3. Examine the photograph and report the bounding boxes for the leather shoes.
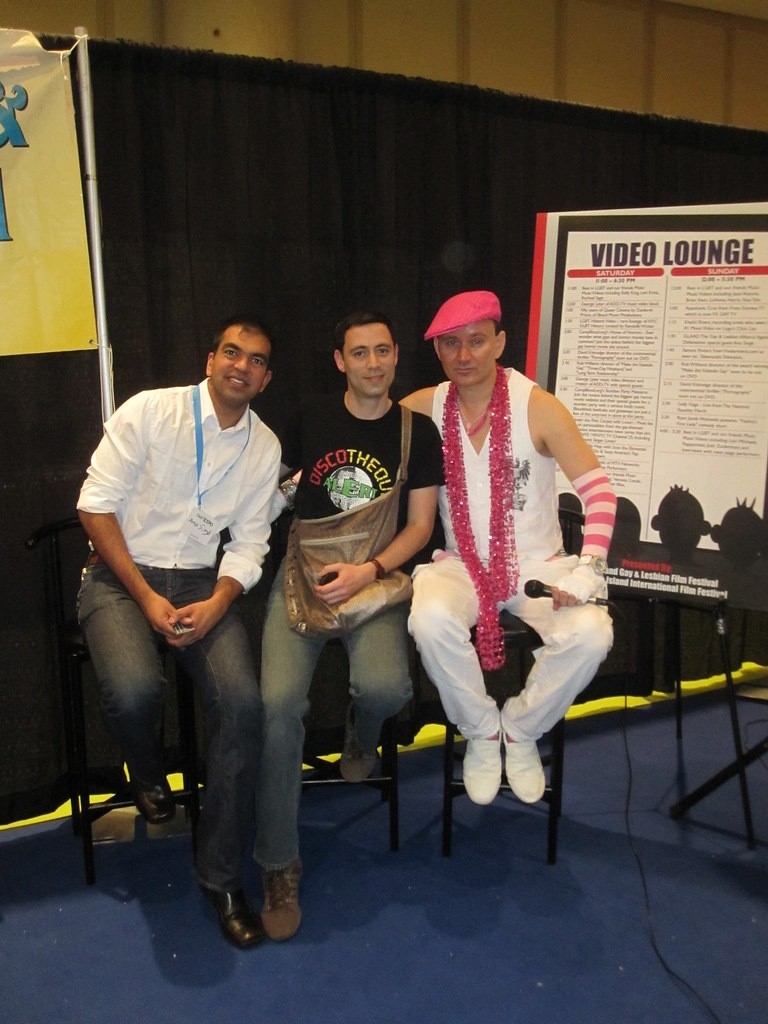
[133,780,175,824]
[462,712,546,805]
[203,888,265,950]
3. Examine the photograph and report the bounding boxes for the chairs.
[29,518,586,887]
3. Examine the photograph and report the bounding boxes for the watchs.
[579,555,607,574]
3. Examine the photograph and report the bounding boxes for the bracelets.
[371,559,384,578]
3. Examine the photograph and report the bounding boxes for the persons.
[254,311,442,941]
[268,291,618,805]
[77,312,283,948]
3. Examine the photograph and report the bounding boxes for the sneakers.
[340,701,377,783]
[260,857,302,941]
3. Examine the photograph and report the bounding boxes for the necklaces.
[443,363,520,671]
[456,397,490,436]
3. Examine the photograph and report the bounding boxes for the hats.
[424,291,501,340]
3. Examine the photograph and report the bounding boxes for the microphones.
[524,580,615,607]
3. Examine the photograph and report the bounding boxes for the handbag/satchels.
[283,404,416,638]
